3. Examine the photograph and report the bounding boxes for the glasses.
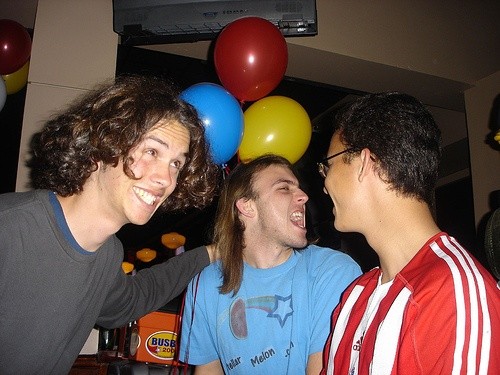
[317,148,376,178]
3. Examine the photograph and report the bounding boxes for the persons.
[178,153,363,375]
[0,73,221,375]
[316,90,500,375]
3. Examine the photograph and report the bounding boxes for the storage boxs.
[129,311,187,367]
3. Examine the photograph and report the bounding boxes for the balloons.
[238,94,313,166]
[177,81,245,170]
[0,18,32,112]
[213,16,290,103]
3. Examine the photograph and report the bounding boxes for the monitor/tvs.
[112,0,318,45]
